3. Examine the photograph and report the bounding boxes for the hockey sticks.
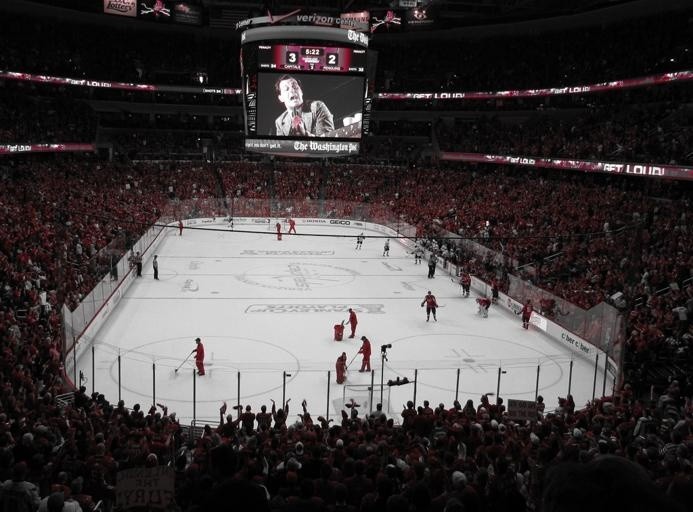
[175,350,195,373]
[345,349,361,370]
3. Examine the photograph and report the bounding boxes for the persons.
[1,81,689,512]
[270,74,338,142]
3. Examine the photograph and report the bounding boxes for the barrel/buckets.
[334,323,344,342]
[278,232,282,241]
